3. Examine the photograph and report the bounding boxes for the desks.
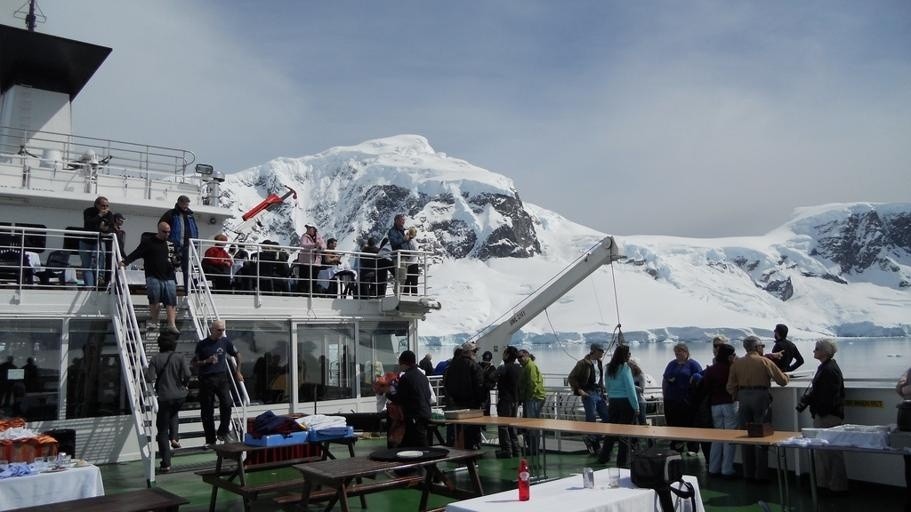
[0,403,910,512]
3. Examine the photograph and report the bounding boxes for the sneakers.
[167,326,180,335]
[513,450,521,458]
[495,450,512,458]
[218,433,236,443]
[202,444,217,451]
[159,466,171,471]
[149,323,161,329]
[526,449,538,455]
[171,440,181,449]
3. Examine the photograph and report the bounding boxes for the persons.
[803,338,848,496]
[599,345,640,468]
[250,340,385,405]
[0,345,132,422]
[117,221,180,333]
[625,353,653,448]
[897,368,910,504]
[193,319,244,450]
[664,344,703,456]
[375,338,543,474]
[727,336,789,489]
[143,334,191,473]
[0,196,421,298]
[713,338,724,365]
[773,324,804,372]
[704,344,744,481]
[568,343,609,454]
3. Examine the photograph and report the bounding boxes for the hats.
[590,342,604,350]
[399,351,415,367]
[305,223,317,228]
[464,343,479,350]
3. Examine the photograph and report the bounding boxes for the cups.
[608,464,621,487]
[58,452,66,465]
[308,425,317,440]
[34,455,57,467]
[347,425,355,435]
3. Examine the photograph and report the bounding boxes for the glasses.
[162,231,170,233]
[755,344,766,349]
[96,203,108,207]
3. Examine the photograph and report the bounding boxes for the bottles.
[519,459,530,502]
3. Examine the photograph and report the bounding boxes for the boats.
[488,369,666,419]
[1,0,910,511]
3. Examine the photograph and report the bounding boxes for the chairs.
[0,225,361,298]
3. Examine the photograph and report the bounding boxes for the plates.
[73,463,92,467]
[396,450,423,458]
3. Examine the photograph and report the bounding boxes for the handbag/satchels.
[631,448,683,489]
